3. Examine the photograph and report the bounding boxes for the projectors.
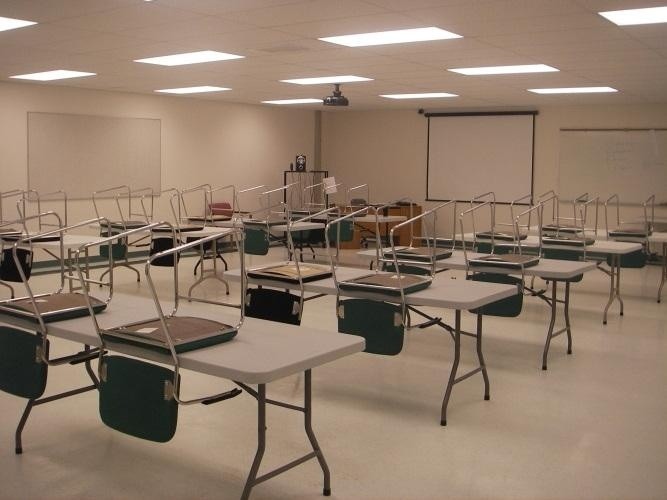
[323,91,349,107]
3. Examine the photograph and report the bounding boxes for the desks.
[183,213,325,275]
[277,210,409,271]
[0,286,366,499]
[6,231,116,301]
[570,225,663,304]
[222,260,519,426]
[357,247,596,371]
[420,232,641,324]
[100,215,234,302]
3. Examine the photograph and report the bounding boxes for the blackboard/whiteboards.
[555,128,667,208]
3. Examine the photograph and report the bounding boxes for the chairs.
[539,191,588,237]
[458,201,543,317]
[1,179,369,261]
[538,197,600,280]
[375,195,457,278]
[468,193,532,254]
[602,194,658,269]
[0,213,111,456]
[326,199,432,355]
[74,222,244,443]
[239,204,340,325]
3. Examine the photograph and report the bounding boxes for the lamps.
[322,83,349,107]
